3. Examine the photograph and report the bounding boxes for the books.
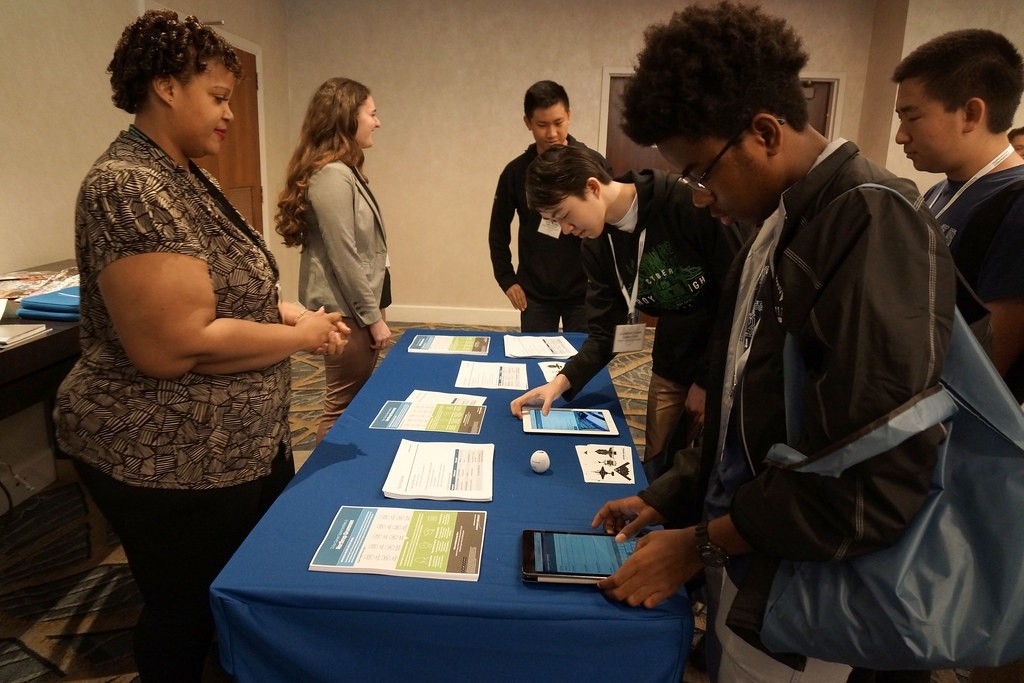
[0,324,54,350]
[17,285,81,321]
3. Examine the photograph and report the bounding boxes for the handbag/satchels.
[725,188,1023,675]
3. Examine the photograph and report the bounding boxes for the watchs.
[694,517,731,571]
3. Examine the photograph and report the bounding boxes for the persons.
[592,1,958,683]
[508,143,747,484]
[892,27,1024,682]
[48,11,355,683]
[1005,125,1023,159]
[274,73,393,447]
[487,79,611,336]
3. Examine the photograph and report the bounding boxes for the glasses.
[677,117,785,197]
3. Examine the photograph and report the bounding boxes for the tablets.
[521,529,645,579]
[521,407,619,436]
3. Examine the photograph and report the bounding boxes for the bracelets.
[293,307,309,323]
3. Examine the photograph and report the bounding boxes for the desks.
[209,329,694,683]
[0,258,110,516]
